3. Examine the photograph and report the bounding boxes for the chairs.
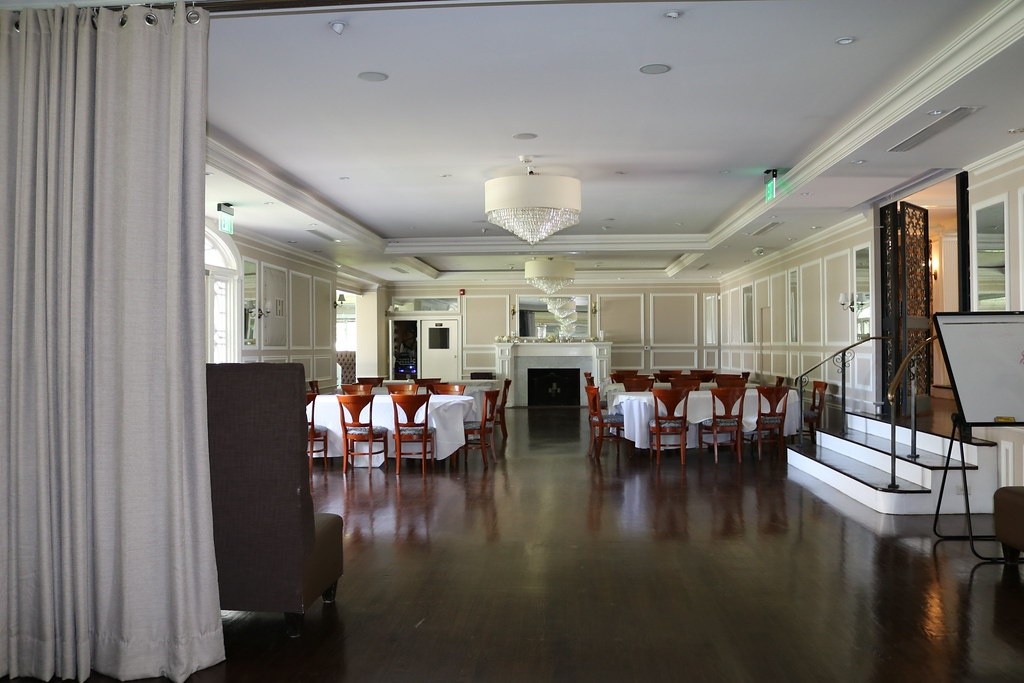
[754,478,789,539]
[708,475,746,540]
[305,368,828,475]
[390,475,438,554]
[587,456,628,534]
[206,364,342,640]
[343,471,389,552]
[653,479,691,543]
[451,463,501,545]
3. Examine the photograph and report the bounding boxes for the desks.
[612,388,805,465]
[603,382,759,436]
[600,377,676,411]
[334,387,491,439]
[381,380,502,411]
[305,394,476,467]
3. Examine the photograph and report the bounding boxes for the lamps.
[538,296,578,338]
[334,294,346,308]
[510,305,516,320]
[484,166,582,245]
[524,256,575,295]
[857,293,866,310]
[591,302,597,316]
[245,301,255,317]
[839,292,855,312]
[258,300,272,319]
[385,305,394,317]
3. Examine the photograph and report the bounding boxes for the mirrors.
[855,241,871,344]
[242,260,256,345]
[516,294,592,340]
[970,192,1010,311]
